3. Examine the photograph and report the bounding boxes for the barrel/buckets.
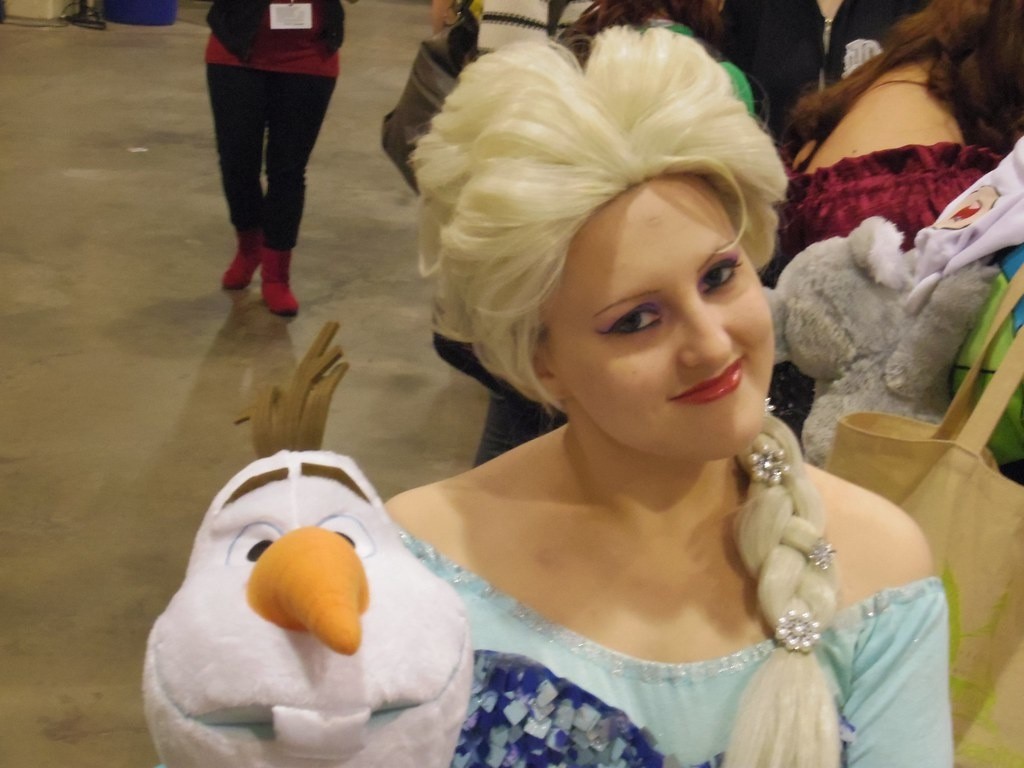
[102,0,178,26]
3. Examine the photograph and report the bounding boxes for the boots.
[222,229,265,289]
[259,244,298,316]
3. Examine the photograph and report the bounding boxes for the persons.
[203,0,351,316]
[382,24,957,768]
[375,0,1024,478]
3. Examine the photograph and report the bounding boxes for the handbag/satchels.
[828,265,1024,768]
[381,0,484,197]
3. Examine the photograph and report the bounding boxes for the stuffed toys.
[762,216,1001,472]
[139,319,471,768]
